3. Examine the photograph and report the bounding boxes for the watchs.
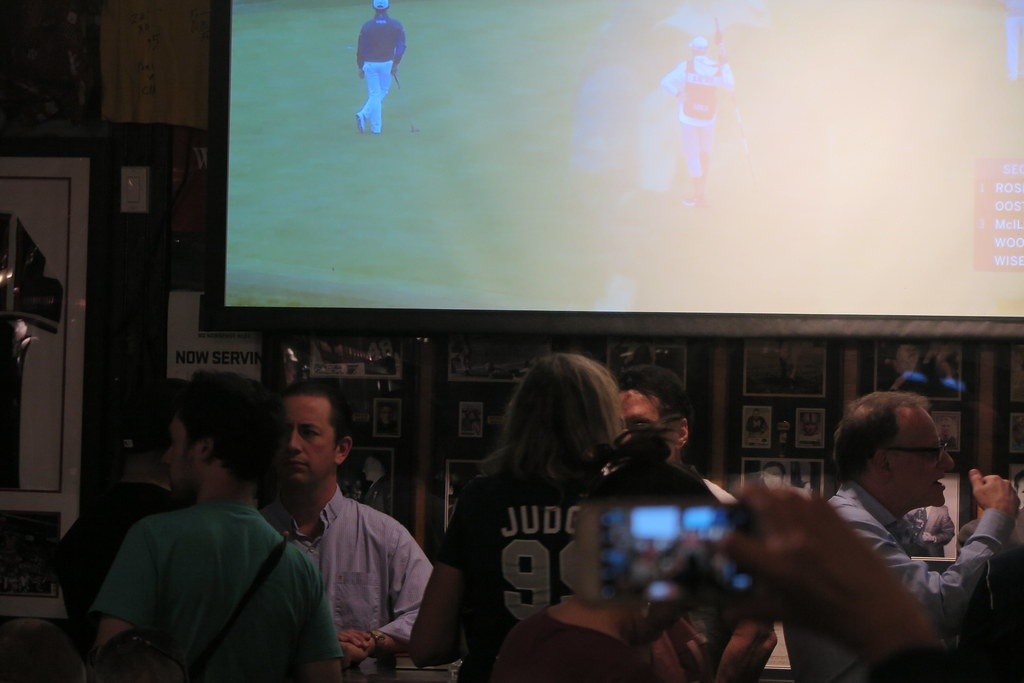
[367,628,386,658]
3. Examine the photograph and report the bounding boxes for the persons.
[461,407,481,435]
[800,411,822,445]
[355,0,405,134]
[747,338,823,393]
[407,352,739,683]
[721,391,1024,683]
[1011,469,1024,546]
[57,369,344,683]
[746,461,803,515]
[889,342,959,399]
[490,423,776,683]
[660,35,735,205]
[378,405,398,434]
[745,408,768,447]
[0,619,190,683]
[258,378,434,666]
[360,454,390,513]
[904,506,954,557]
[939,417,957,447]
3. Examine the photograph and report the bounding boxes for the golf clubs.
[395,73,420,133]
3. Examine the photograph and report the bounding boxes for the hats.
[372,0,389,10]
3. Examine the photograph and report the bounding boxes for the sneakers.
[370,125,380,133]
[356,112,366,133]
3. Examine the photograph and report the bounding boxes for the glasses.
[884,442,948,461]
[88,630,188,683]
[621,415,684,432]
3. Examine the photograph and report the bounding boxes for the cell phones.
[576,502,761,606]
